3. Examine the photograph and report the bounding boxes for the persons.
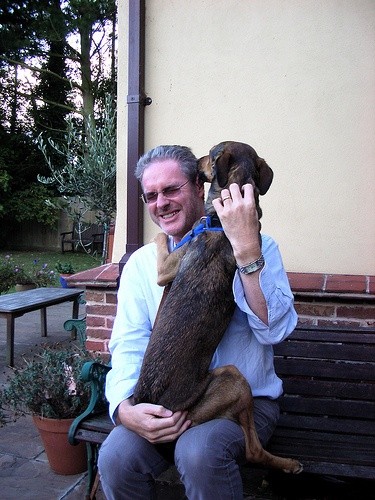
[98,145,298,499]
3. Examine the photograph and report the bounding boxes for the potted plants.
[56,261,78,289]
[0,342,112,477]
[0,255,37,294]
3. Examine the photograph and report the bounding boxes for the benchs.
[63,294,87,354]
[68,328,375,500]
[60,222,104,254]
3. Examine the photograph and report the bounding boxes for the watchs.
[237,254,266,274]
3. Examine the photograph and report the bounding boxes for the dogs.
[131,138,305,479]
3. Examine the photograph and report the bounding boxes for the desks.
[0,287,84,367]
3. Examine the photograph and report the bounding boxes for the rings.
[220,196,232,203]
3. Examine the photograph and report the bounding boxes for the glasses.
[141,179,191,204]
